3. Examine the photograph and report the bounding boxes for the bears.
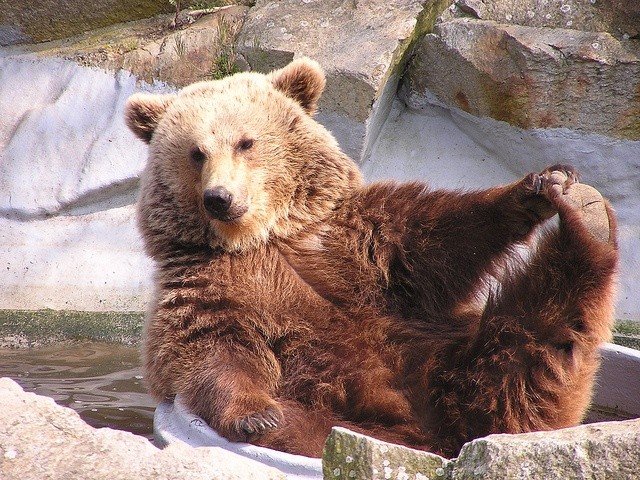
[122,56,620,462]
